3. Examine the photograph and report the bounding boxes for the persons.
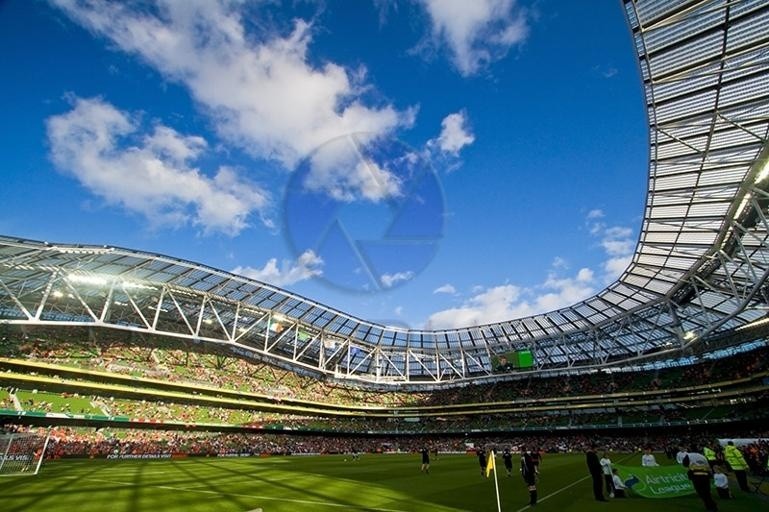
[519,445,541,509]
[712,464,736,500]
[586,443,610,503]
[239,355,442,457]
[476,446,487,478]
[600,452,617,495]
[439,346,768,453]
[351,446,362,461]
[433,445,439,460]
[1,321,252,472]
[641,449,661,467]
[503,448,513,475]
[725,440,753,493]
[422,443,431,473]
[682,443,718,512]
[608,469,631,499]
